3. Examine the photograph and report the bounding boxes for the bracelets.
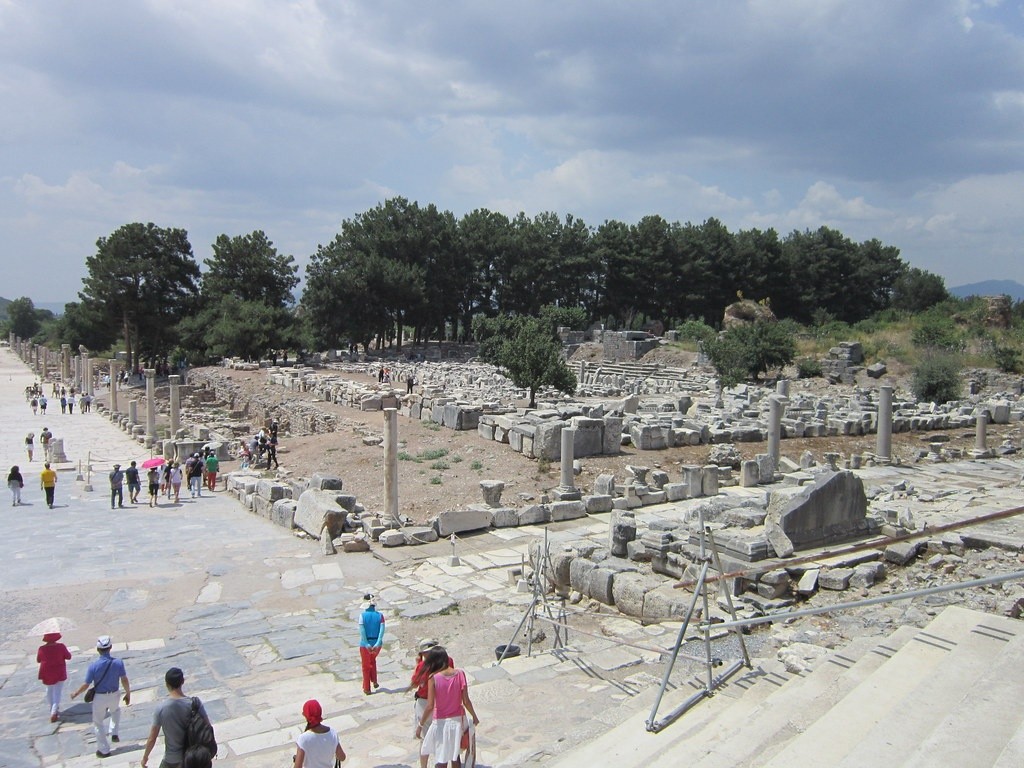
[418,722,423,728]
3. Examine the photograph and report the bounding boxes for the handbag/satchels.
[84,687,95,702]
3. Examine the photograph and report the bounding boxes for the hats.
[360,594,377,612]
[43,633,61,642]
[194,453,200,457]
[96,634,111,649]
[302,700,322,726]
[418,638,435,654]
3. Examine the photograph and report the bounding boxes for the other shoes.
[373,683,379,688]
[111,735,119,742]
[364,690,371,695]
[133,497,138,502]
[95,749,110,757]
[50,715,60,722]
[130,499,134,504]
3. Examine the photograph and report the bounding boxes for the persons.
[350,343,358,356]
[404,637,480,768]
[40,427,53,461]
[70,635,130,759]
[141,667,217,768]
[379,366,391,384]
[109,464,124,510]
[407,369,415,394]
[358,593,385,695]
[51,383,91,415]
[126,461,142,504]
[272,349,288,366]
[7,465,24,506]
[102,361,177,388]
[23,382,48,416]
[24,432,35,463]
[239,419,280,471]
[292,699,346,768]
[146,459,183,508]
[36,633,72,723]
[39,462,58,509]
[186,446,220,499]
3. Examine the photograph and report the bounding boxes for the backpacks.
[179,696,218,768]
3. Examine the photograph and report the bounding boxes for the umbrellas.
[141,458,165,470]
[26,616,79,638]
